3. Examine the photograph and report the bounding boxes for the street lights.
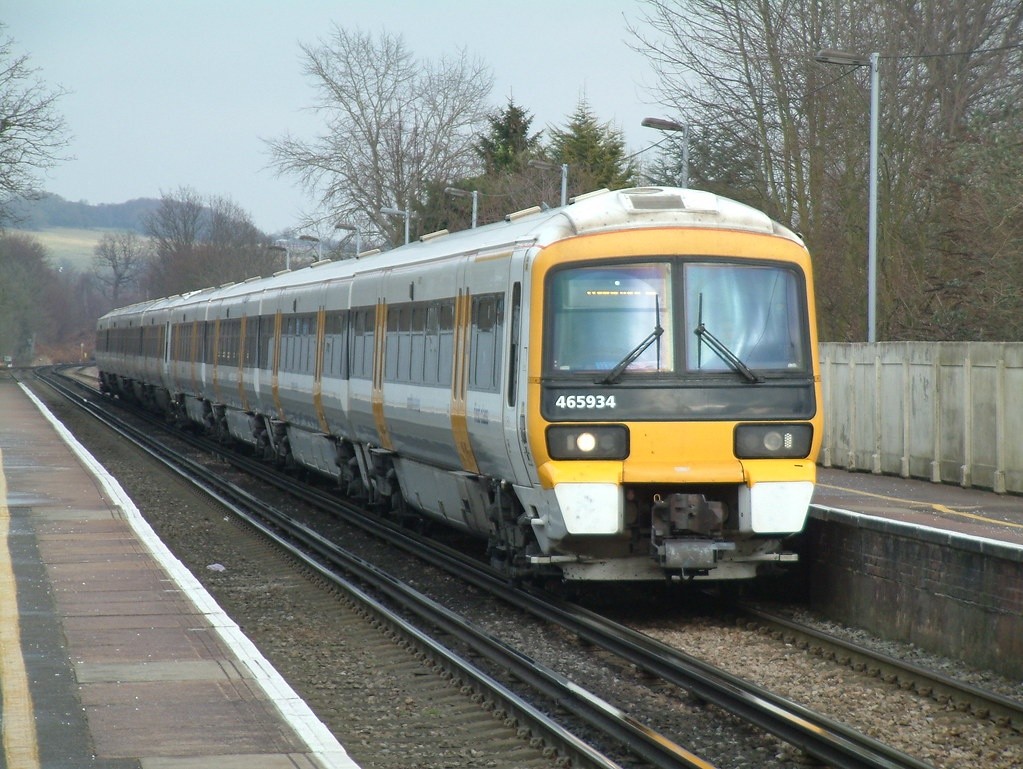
[270,245,289,269]
[335,224,360,255]
[299,235,321,261]
[641,118,689,187]
[380,207,409,245]
[527,160,567,205]
[815,49,880,343]
[444,187,477,228]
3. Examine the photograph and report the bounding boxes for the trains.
[96,186,822,580]
